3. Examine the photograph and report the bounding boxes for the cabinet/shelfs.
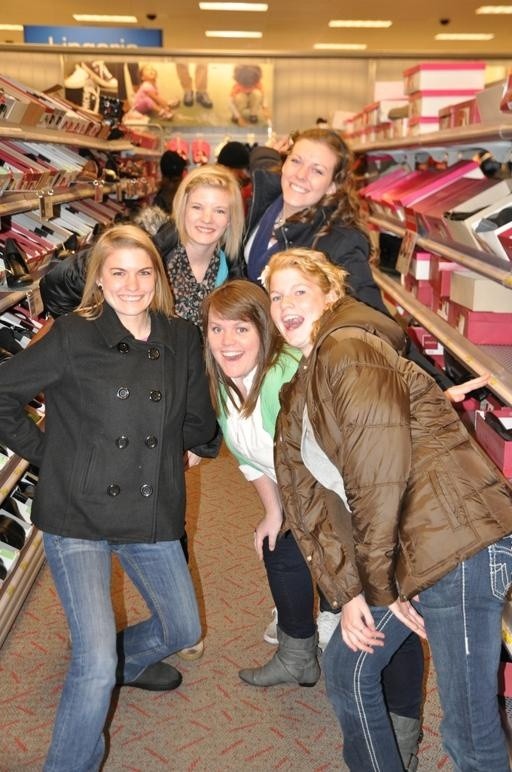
[350,118,512,408]
[0,122,159,653]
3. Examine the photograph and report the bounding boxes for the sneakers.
[64,65,90,90]
[231,115,259,124]
[184,92,213,108]
[79,84,101,113]
[157,100,181,122]
[120,659,183,691]
[177,640,204,661]
[168,138,229,165]
[315,595,342,652]
[81,61,118,89]
[264,608,279,645]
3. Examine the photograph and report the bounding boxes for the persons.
[201,281,427,772]
[0,223,217,771]
[263,246,512,772]
[123,62,269,128]
[36,130,488,660]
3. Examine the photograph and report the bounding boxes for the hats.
[158,150,185,178]
[217,141,250,168]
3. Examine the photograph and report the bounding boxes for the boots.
[390,711,423,771]
[236,623,321,688]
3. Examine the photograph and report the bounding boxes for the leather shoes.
[4,237,34,289]
[443,351,488,401]
[484,412,511,442]
